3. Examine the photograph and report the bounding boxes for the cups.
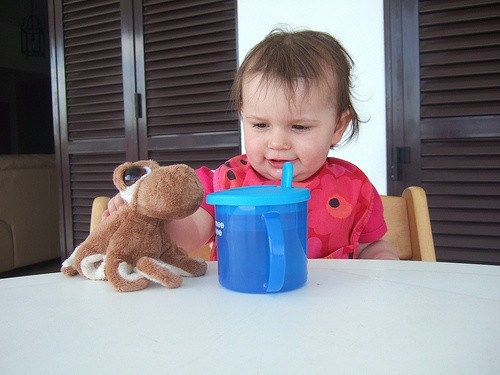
[205,161,310,293]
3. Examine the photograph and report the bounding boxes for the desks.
[0,259,500,375]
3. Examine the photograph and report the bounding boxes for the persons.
[103,27,401,260]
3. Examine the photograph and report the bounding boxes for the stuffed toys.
[60,160,208,292]
